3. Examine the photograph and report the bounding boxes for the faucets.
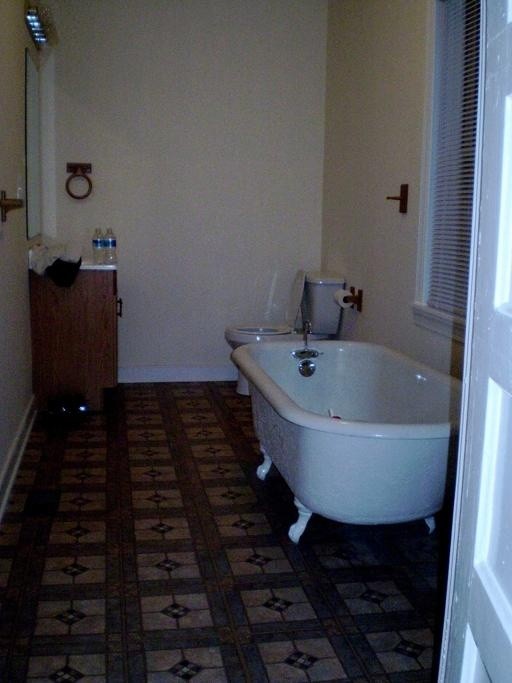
[303,319,313,340]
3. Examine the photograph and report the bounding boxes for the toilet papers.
[334,289,352,308]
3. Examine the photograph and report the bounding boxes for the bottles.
[78,226,118,266]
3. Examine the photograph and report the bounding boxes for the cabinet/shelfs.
[29,269,122,413]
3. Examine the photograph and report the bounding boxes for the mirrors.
[25,48,40,240]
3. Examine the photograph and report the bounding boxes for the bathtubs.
[231,340,462,543]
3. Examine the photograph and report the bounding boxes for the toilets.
[225,271,345,395]
